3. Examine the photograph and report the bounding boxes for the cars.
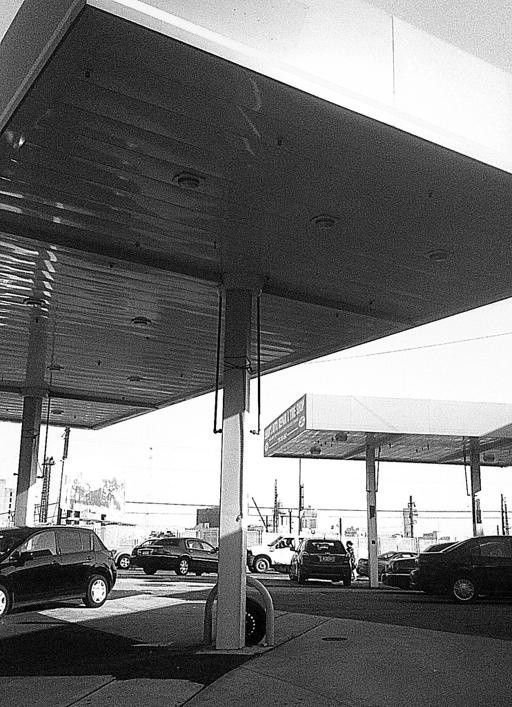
[246,536,352,586]
[0,525,219,618]
[356,535,512,602]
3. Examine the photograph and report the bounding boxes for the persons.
[346,540,358,581]
